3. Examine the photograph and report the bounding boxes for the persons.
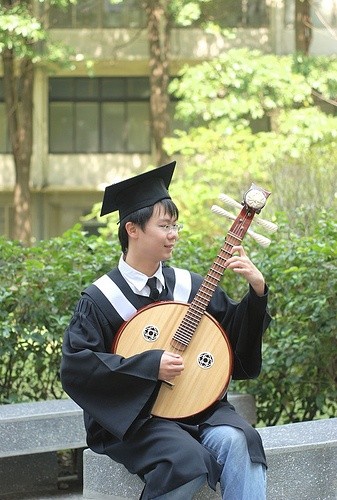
[57,159,274,500]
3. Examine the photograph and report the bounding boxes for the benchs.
[0,391,337,500]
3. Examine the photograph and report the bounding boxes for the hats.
[100,160,177,225]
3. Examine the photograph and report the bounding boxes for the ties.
[145,276,160,302]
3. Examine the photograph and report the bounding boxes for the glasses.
[143,222,181,232]
